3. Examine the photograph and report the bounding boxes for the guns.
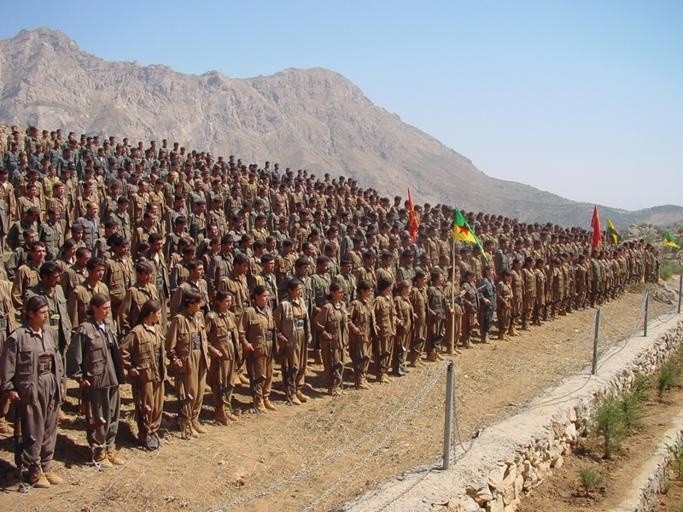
[14,162,660,493]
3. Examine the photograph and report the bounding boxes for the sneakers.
[254,397,276,415]
[234,372,252,388]
[179,418,208,439]
[59,409,72,424]
[374,274,663,384]
[285,389,310,405]
[205,382,214,392]
[29,467,63,489]
[0,413,15,435]
[313,350,325,365]
[354,372,373,391]
[328,389,348,397]
[214,408,238,426]
[95,452,126,473]
[138,430,166,450]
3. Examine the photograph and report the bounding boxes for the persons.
[2,125,664,488]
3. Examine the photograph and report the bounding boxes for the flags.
[663,231,679,249]
